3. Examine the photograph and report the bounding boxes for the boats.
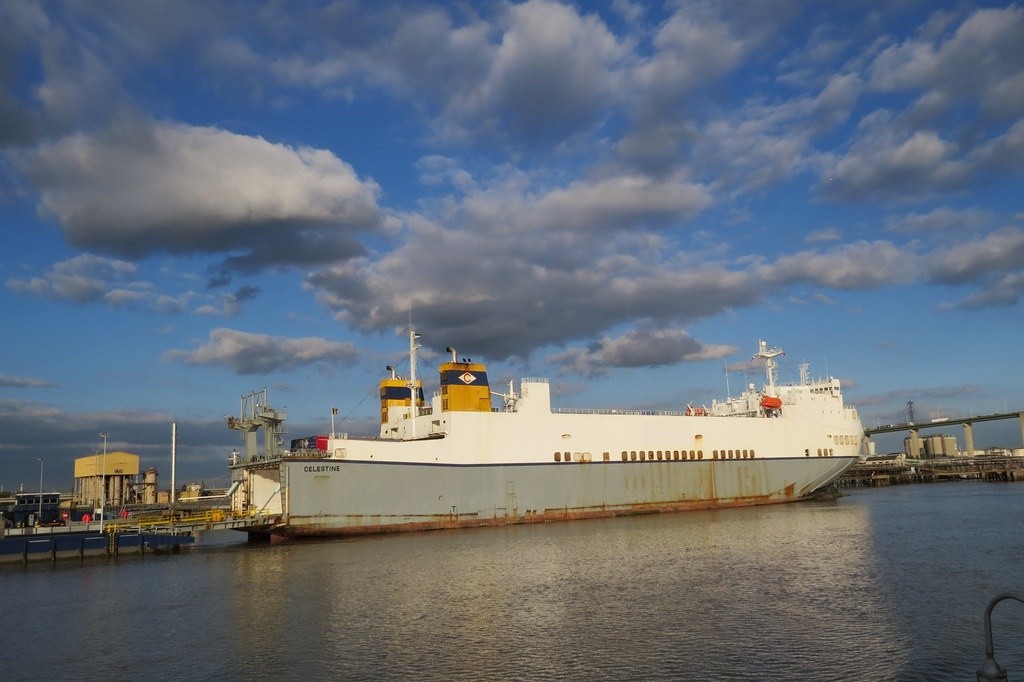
[0,425,198,566]
[761,398,782,409]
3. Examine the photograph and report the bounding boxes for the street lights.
[100,433,111,533]
[38,457,44,516]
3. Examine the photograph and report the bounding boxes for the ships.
[215,302,864,536]
